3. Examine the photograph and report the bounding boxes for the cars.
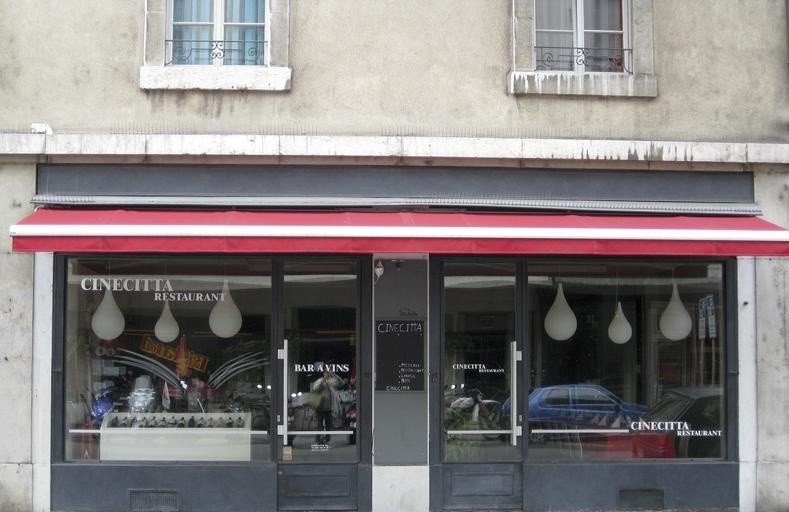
[502,384,725,459]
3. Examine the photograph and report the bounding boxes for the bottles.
[110,415,244,428]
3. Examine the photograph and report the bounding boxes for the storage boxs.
[97,412,252,463]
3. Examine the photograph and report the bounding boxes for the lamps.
[208,260,243,338]
[606,258,634,344]
[90,261,125,341]
[153,264,180,343]
[542,259,579,342]
[659,256,693,342]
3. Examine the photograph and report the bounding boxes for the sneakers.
[326,442,331,449]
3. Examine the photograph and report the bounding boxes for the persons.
[311,366,345,448]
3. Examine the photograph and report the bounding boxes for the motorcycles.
[443,386,502,440]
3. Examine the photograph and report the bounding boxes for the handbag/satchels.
[302,393,322,409]
